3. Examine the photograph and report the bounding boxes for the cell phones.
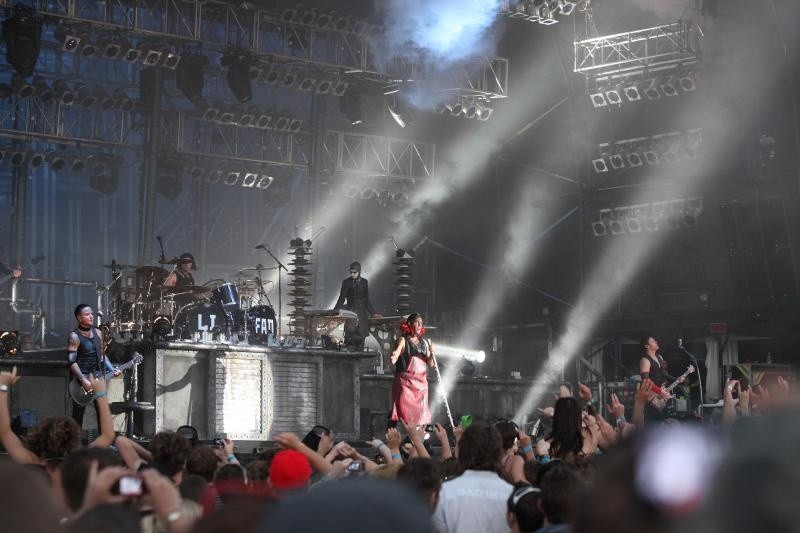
[347,459,361,472]
[422,424,437,433]
[213,438,228,445]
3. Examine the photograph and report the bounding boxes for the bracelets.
[164,511,185,529]
[725,385,733,391]
[616,416,625,421]
[95,391,107,398]
[377,443,384,450]
[392,453,401,459]
[227,456,235,462]
[522,445,532,453]
[584,399,595,406]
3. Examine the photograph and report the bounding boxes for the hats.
[407,313,421,320]
[270,450,311,488]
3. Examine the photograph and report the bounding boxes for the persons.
[328,262,383,351]
[0,365,800,533]
[640,335,686,427]
[67,304,122,452]
[163,252,197,339]
[385,313,434,445]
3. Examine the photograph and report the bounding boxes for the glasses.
[350,271,357,273]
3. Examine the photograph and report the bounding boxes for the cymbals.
[243,267,277,270]
[135,266,170,278]
[181,285,211,293]
[159,259,193,264]
[103,264,137,271]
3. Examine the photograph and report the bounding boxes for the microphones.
[96,308,105,318]
[676,336,684,350]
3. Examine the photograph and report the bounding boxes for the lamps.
[477,350,497,363]
[0,0,705,237]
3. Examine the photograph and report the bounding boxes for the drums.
[212,283,239,308]
[118,302,144,333]
[241,305,277,345]
[203,279,227,292]
[174,302,228,341]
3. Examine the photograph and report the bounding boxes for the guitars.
[651,365,695,412]
[68,353,144,406]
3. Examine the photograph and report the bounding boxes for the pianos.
[304,308,358,349]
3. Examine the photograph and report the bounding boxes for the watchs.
[0,384,9,392]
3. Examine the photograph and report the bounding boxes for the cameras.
[117,471,144,498]
